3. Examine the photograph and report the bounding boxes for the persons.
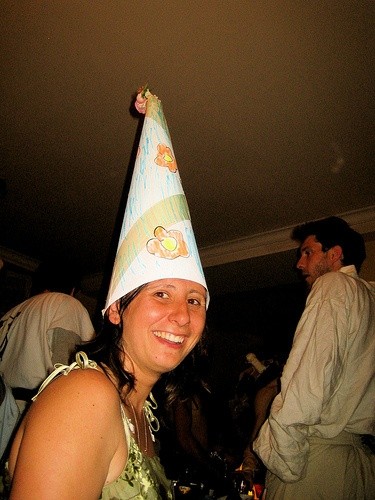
[174,351,283,475]
[251,216,375,500]
[0,252,209,500]
[0,256,96,500]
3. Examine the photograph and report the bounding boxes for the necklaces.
[121,385,148,455]
